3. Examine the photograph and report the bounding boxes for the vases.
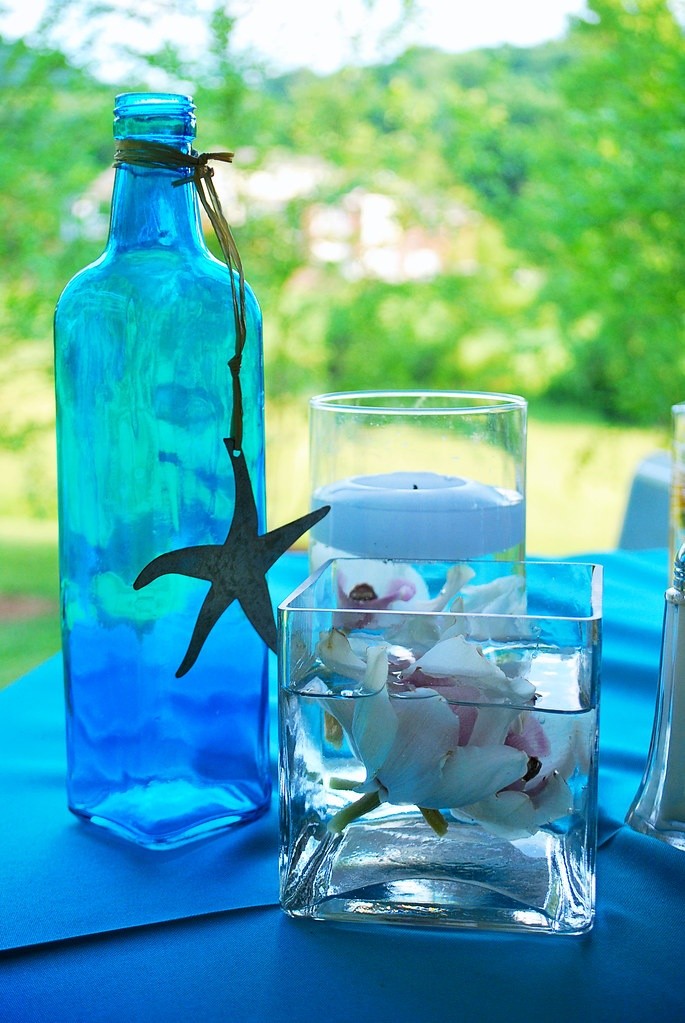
[276,556,606,936]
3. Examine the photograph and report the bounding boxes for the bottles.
[53,93,274,851]
[625,540,685,851]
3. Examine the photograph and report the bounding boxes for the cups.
[307,392,526,570]
[277,557,603,935]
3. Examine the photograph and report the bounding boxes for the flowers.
[283,558,596,839]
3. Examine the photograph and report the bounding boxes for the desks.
[1,539,685,1023]
